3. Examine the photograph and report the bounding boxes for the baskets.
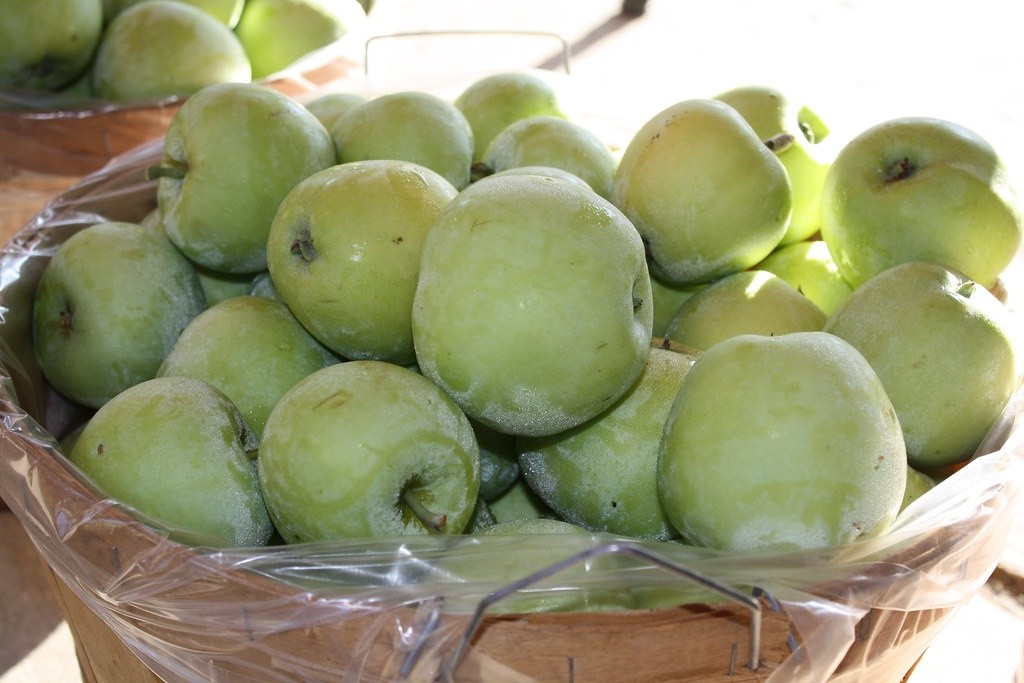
[0,32,1024,683]
[0,0,368,257]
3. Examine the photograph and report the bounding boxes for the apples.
[26,70,1023,614]
[0,0,373,111]
